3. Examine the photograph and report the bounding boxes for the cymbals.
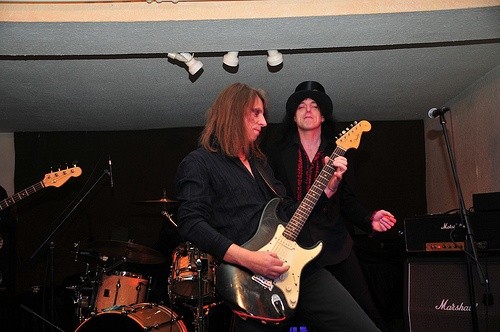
[130,198,178,206]
[87,240,165,265]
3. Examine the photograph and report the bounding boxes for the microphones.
[427,107,449,119]
[108,153,114,188]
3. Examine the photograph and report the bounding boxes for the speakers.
[406,257,500,332]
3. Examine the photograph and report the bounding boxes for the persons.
[268,80,397,318]
[177,83,380,332]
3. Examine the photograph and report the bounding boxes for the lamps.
[267,50,283,66]
[168,52,203,75]
[223,51,239,66]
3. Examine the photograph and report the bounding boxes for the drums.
[73,303,188,332]
[169,243,216,299]
[93,270,152,313]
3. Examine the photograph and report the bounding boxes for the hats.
[286,81,333,122]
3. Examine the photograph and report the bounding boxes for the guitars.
[213,119,372,320]
[0,163,83,212]
